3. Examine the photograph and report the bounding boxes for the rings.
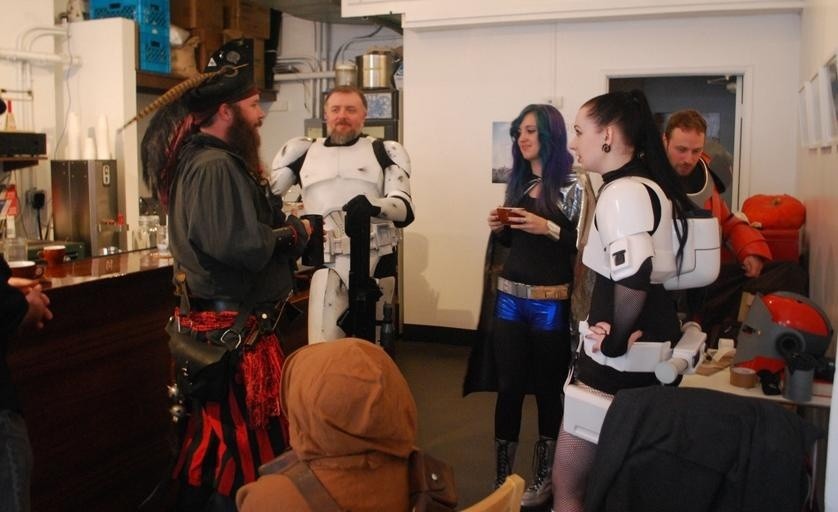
[522,218,527,223]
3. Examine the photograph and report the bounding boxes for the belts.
[495,276,572,302]
[175,297,251,312]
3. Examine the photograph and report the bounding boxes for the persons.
[551,88,725,512]
[640,107,777,361]
[268,85,416,354]
[453,104,592,509]
[158,34,328,511]
[231,338,460,512]
[0,251,58,346]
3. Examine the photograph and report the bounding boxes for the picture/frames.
[796,52,838,151]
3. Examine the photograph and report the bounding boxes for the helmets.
[731,290,833,380]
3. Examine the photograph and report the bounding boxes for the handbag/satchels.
[168,332,231,402]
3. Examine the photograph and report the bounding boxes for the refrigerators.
[318,89,405,339]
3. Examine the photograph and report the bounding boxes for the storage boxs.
[171,0,271,90]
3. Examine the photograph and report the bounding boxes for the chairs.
[586,384,820,511]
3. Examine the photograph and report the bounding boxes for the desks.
[676,342,832,512]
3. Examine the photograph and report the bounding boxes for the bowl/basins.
[496,207,527,226]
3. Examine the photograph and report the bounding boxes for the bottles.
[4,99,17,132]
[132,214,162,252]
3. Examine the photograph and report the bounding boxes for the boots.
[493,437,519,491]
[520,434,556,511]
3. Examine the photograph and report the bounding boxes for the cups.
[43,245,67,265]
[6,260,45,280]
[156,224,169,250]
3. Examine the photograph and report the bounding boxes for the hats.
[182,38,254,113]
